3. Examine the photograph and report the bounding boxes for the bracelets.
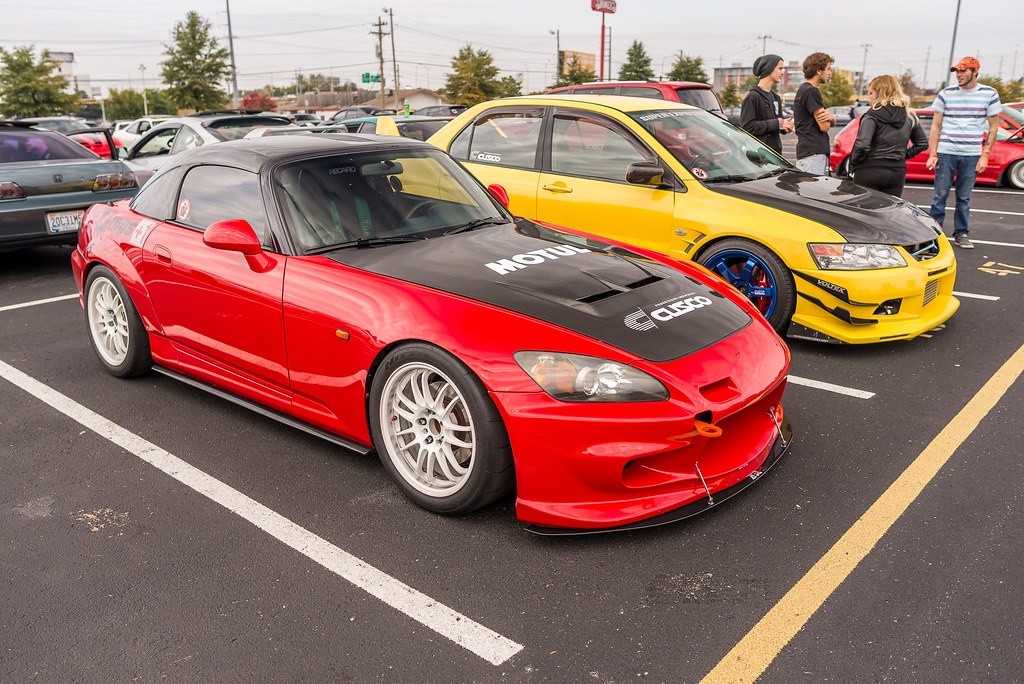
[930,154,937,157]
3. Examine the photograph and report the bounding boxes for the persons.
[854,99,862,116]
[850,75,929,198]
[741,54,794,155]
[792,53,836,175]
[925,57,1002,249]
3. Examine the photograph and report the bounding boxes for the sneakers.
[954,233,975,248]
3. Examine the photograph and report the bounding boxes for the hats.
[950,57,980,73]
[752,55,782,79]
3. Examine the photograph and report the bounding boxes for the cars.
[373,95,961,347]
[123,109,349,190]
[830,103,1024,190]
[723,107,741,126]
[0,117,141,250]
[21,106,472,158]
[71,133,794,535]
[826,105,852,126]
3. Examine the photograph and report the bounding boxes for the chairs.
[601,119,649,163]
[556,133,579,169]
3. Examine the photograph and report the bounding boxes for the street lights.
[139,64,149,116]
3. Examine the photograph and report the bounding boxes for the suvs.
[501,77,731,171]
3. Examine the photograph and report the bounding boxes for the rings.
[929,167,932,170]
